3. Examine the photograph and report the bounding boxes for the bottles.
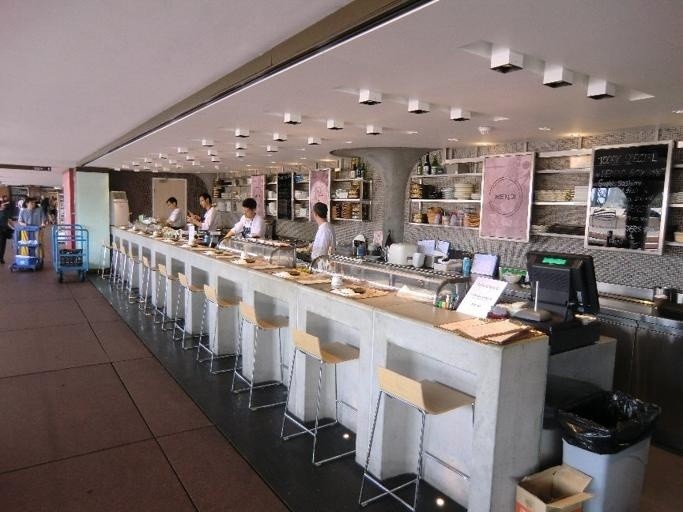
[430,155,441,175]
[416,158,422,175]
[422,152,430,175]
[349,157,367,180]
[433,209,469,228]
[462,256,471,274]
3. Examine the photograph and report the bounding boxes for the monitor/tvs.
[509,250,600,337]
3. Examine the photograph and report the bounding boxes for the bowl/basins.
[503,274,521,284]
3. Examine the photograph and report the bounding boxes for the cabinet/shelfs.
[213,171,372,222]
[407,136,683,247]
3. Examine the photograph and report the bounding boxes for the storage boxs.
[513,464,594,512]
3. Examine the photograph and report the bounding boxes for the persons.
[223,197,266,244]
[186,193,218,233]
[0,194,57,263]
[310,203,336,262]
[166,197,184,230]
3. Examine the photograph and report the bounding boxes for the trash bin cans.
[555,390,662,512]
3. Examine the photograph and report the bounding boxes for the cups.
[329,274,343,289]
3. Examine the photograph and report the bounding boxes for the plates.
[440,182,481,200]
[529,185,683,242]
[210,174,367,220]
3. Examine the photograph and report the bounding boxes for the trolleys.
[8,224,42,272]
[52,224,89,283]
